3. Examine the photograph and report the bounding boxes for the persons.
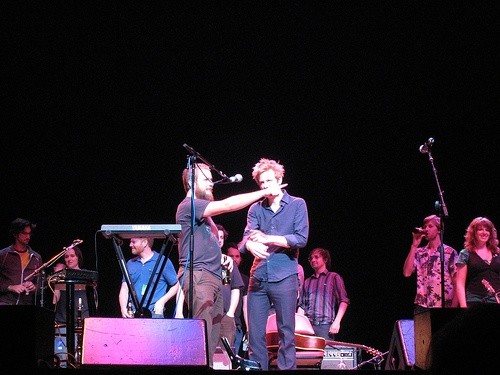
[0,219,44,306]
[176,162,288,357]
[456,216,500,308]
[118,238,180,319]
[243,159,309,371]
[176,224,243,354]
[52,245,90,365]
[403,215,458,313]
[297,248,349,340]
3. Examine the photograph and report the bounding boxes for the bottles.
[128,300,133,318]
[414,229,427,235]
[221,257,230,285]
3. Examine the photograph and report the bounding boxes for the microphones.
[419,137,435,153]
[413,227,428,236]
[111,234,123,246]
[217,174,243,184]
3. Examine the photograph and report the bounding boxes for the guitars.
[263,333,383,358]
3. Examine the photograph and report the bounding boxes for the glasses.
[308,256,321,262]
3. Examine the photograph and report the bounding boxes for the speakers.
[81,316,208,368]
[412,308,500,375]
[318,344,358,369]
[384,320,417,371]
[0,305,55,375]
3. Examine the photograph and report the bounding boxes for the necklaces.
[15,248,28,253]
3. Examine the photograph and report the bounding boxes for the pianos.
[99,223,182,321]
